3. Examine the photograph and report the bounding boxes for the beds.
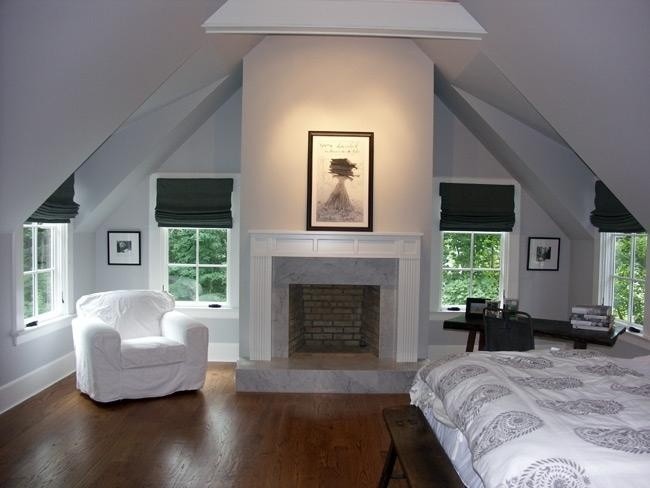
[409,351,650,487]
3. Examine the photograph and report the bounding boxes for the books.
[570,304,616,332]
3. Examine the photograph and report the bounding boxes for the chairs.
[482,308,534,350]
[72,289,209,403]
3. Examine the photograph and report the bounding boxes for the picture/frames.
[306,132,374,232]
[527,237,560,271]
[107,231,141,265]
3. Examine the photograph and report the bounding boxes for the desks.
[443,313,626,352]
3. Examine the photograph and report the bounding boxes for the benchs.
[379,405,466,487]
